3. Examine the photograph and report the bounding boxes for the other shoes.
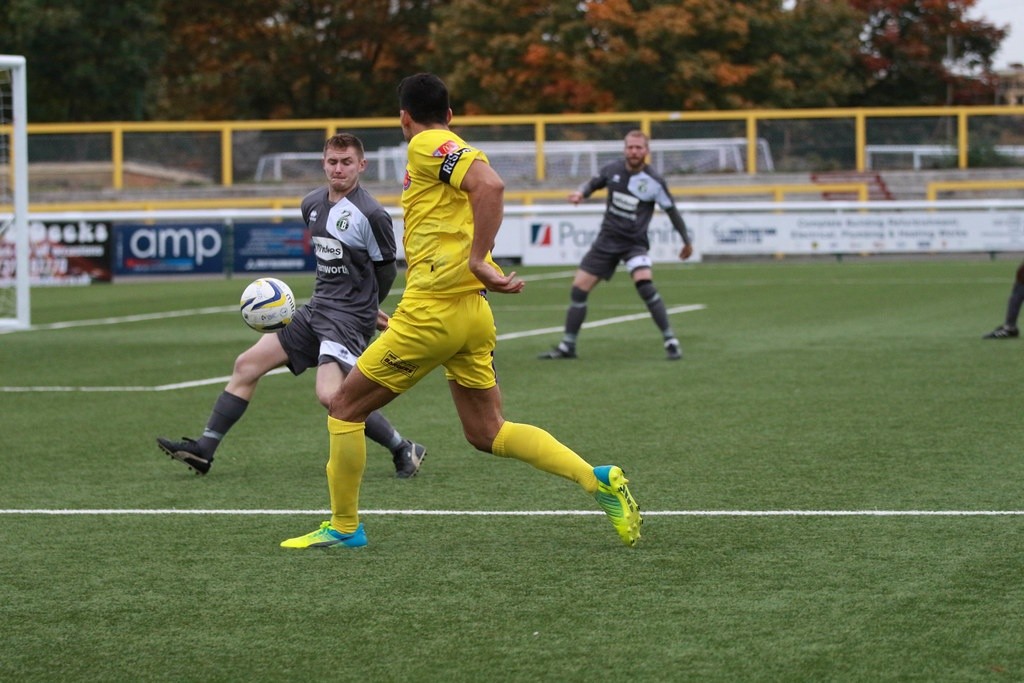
[663,336,682,361]
[535,342,576,359]
[982,325,1020,340]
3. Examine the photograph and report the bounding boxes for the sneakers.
[392,439,428,480]
[593,464,644,547]
[279,519,368,549]
[156,436,214,476]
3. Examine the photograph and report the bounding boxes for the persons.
[280,72,643,549]
[536,131,692,359]
[983,258,1024,339]
[156,134,427,477]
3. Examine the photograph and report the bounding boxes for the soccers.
[239,276,297,333]
